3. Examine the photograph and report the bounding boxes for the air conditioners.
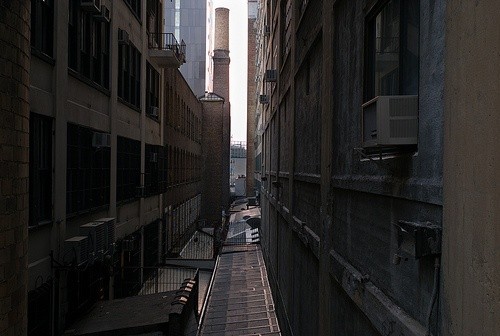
[92,217,116,250]
[361,95,418,154]
[265,69,277,82]
[258,95,269,104]
[93,5,111,23]
[262,25,271,38]
[118,28,130,47]
[150,106,160,117]
[101,133,112,148]
[89,131,104,148]
[81,0,101,15]
[63,235,90,268]
[78,221,106,256]
